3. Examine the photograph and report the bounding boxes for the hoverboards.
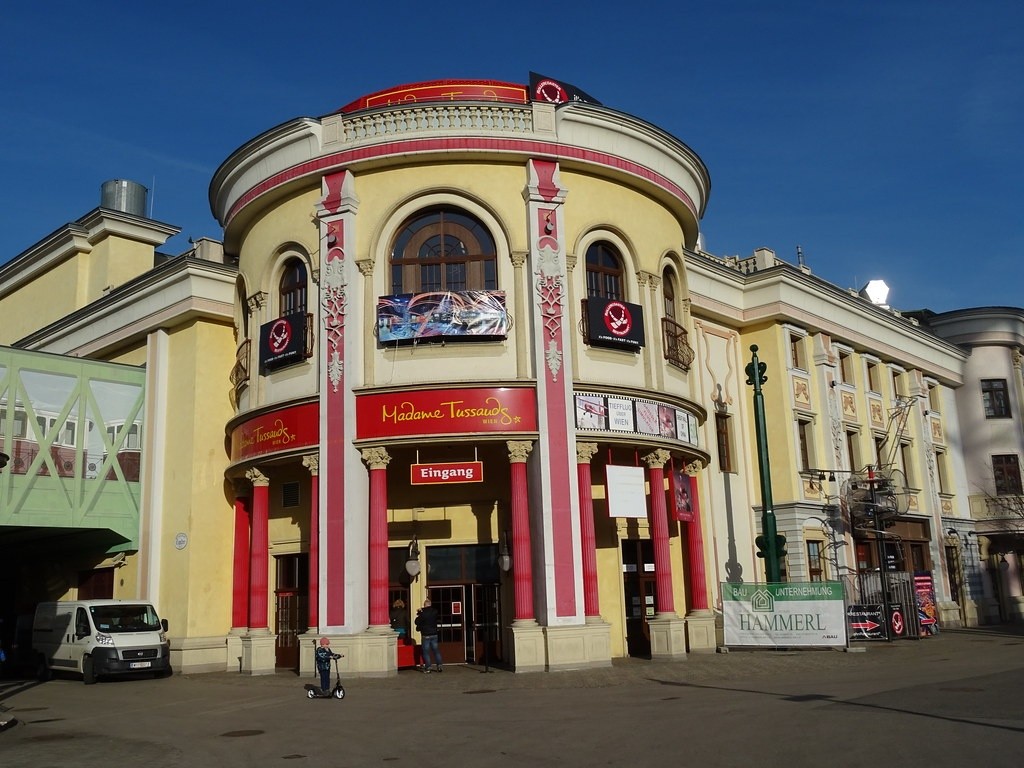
[303,655,346,700]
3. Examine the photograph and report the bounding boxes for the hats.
[320,636,330,645]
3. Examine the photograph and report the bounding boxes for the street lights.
[744,342,783,583]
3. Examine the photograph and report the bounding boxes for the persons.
[390,599,408,638]
[316,637,343,694]
[414,598,444,674]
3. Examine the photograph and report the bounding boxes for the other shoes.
[323,690,332,694]
[426,666,432,673]
[438,664,443,672]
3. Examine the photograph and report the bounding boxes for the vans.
[30,597,172,685]
